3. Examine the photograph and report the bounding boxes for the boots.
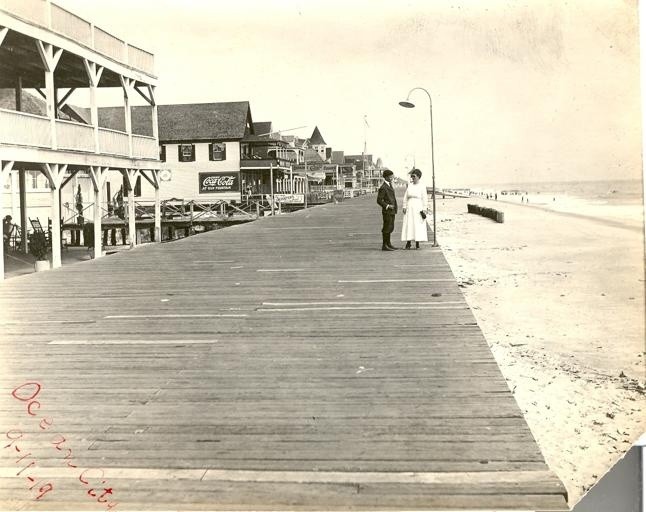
[382,233,399,251]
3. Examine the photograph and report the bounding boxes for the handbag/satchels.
[420,212,426,219]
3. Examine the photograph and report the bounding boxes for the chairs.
[3,216,68,253]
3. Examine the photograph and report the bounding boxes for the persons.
[376,170,399,251]
[401,169,429,249]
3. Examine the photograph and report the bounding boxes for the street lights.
[399,86,437,250]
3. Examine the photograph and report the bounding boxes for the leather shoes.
[406,243,420,249]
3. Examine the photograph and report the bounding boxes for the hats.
[383,170,393,177]
